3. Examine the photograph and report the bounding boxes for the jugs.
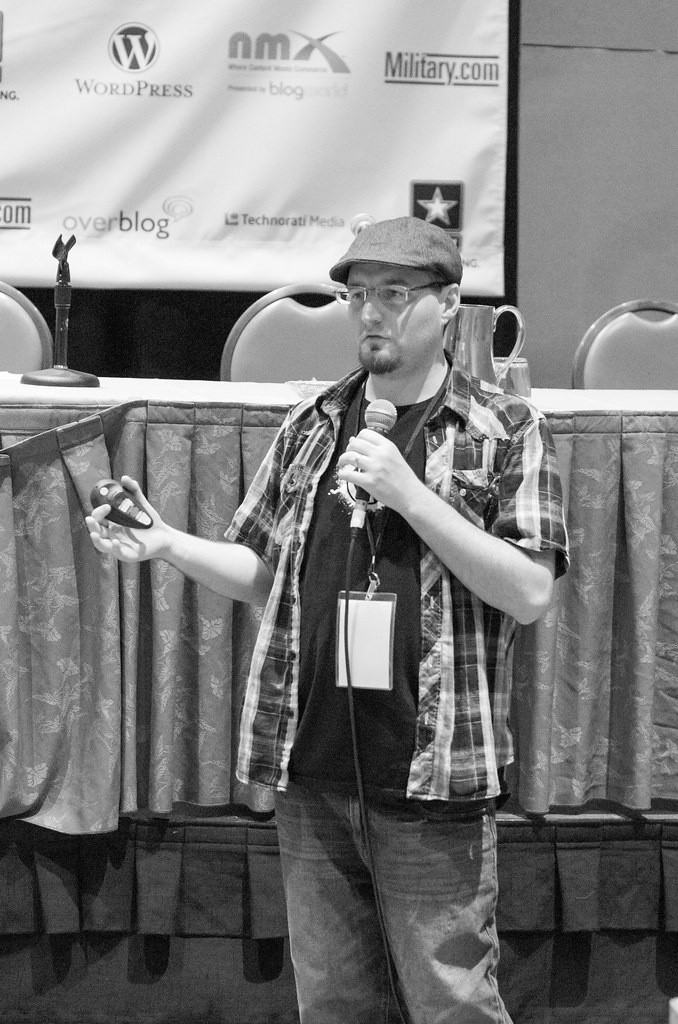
[443,301,527,388]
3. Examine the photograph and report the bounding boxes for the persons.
[85,217,571,1024]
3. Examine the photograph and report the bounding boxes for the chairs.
[572,299,678,390]
[220,282,362,383]
[0,280,56,374]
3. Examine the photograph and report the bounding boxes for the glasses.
[332,281,441,305]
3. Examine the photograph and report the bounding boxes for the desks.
[0,372,678,836]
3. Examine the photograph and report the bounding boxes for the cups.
[493,357,530,397]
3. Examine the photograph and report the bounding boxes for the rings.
[355,453,362,468]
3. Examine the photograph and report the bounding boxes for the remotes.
[91,479,154,529]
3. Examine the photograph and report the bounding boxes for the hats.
[329,216,463,285]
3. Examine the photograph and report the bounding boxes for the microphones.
[350,399,397,538]
